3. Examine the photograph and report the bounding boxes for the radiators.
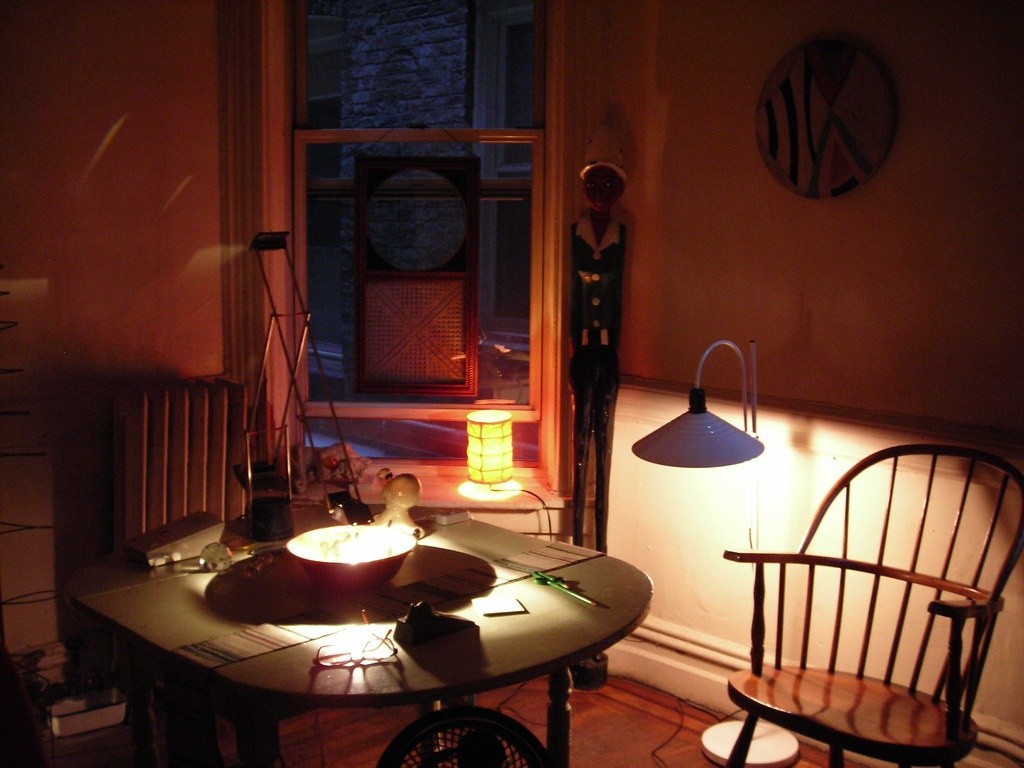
[65,502,653,768]
[119,377,249,548]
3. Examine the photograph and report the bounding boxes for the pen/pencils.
[531,572,597,607]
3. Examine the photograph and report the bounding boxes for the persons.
[569,125,629,659]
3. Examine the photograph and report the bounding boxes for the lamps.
[467,409,513,484]
[632,339,800,768]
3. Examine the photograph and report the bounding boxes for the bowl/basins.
[284,525,419,601]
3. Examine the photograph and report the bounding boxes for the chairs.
[723,441,1024,768]
